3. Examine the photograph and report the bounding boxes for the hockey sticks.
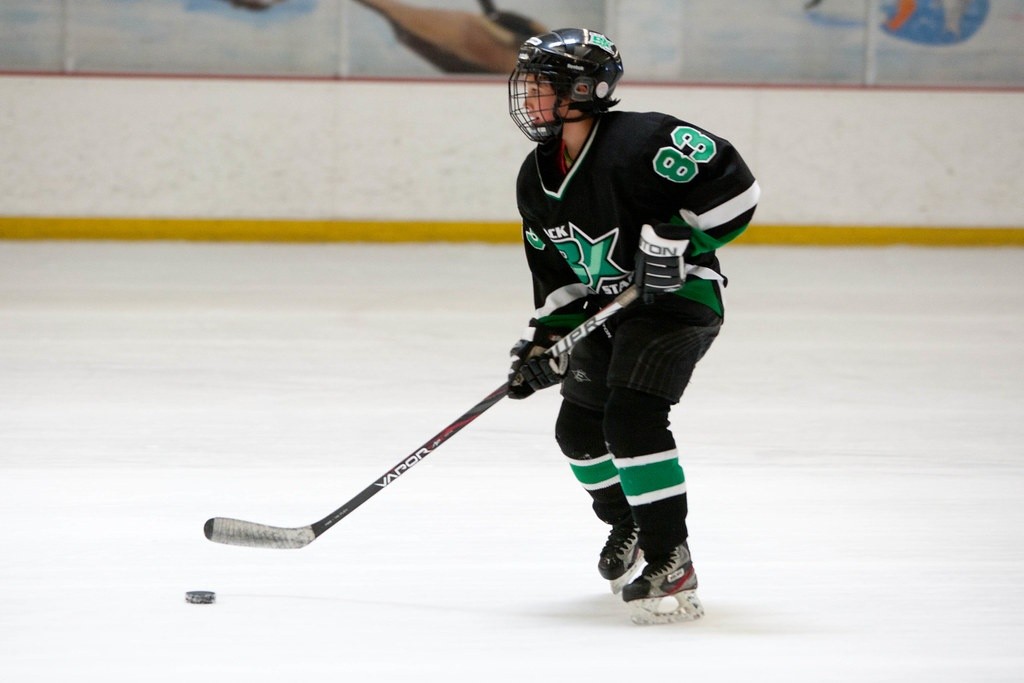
[203,283,640,550]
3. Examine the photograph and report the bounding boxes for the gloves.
[633,222,693,305]
[506,339,571,400]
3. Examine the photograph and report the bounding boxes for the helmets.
[506,27,622,144]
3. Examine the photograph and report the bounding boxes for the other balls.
[185,589,215,605]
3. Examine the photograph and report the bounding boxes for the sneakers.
[591,500,701,625]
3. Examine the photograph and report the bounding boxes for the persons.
[507,28,761,625]
